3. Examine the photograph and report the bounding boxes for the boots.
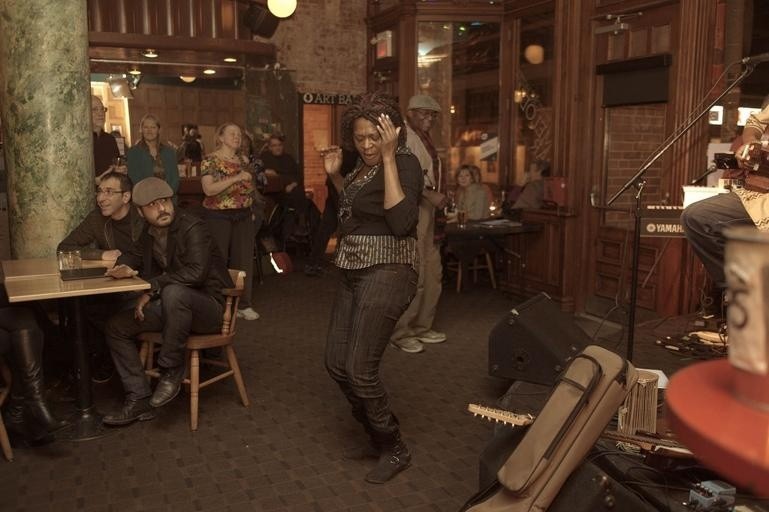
[3,334,49,448]
[13,327,71,434]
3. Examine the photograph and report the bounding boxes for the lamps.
[126,68,143,89]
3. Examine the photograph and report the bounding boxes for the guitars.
[468,403,693,458]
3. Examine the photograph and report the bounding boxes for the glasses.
[93,188,124,197]
[415,110,440,119]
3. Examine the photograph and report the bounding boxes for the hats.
[406,94,442,112]
[131,176,174,207]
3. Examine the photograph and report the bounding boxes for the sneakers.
[415,329,450,342]
[92,361,117,382]
[390,338,427,352]
[236,307,261,322]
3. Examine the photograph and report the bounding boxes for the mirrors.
[512,10,554,187]
[417,20,502,185]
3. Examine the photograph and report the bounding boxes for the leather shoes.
[101,399,156,425]
[342,440,382,461]
[150,364,185,408]
[365,451,412,484]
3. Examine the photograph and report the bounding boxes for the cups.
[56,250,83,277]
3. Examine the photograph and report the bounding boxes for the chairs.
[445,243,497,294]
[277,190,314,254]
[135,268,250,430]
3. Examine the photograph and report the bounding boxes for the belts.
[422,184,438,194]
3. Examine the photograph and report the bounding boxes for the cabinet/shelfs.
[497,207,561,303]
[178,175,284,221]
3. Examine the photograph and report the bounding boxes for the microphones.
[743,52,769,65]
[691,164,717,184]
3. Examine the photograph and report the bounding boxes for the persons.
[128,115,180,208]
[447,165,489,225]
[242,134,268,249]
[471,166,494,217]
[9,299,69,455]
[302,145,359,277]
[202,123,261,321]
[101,176,236,426]
[681,101,769,297]
[391,96,448,353]
[54,172,147,260]
[319,93,424,485]
[92,94,130,183]
[179,122,206,164]
[257,135,323,241]
